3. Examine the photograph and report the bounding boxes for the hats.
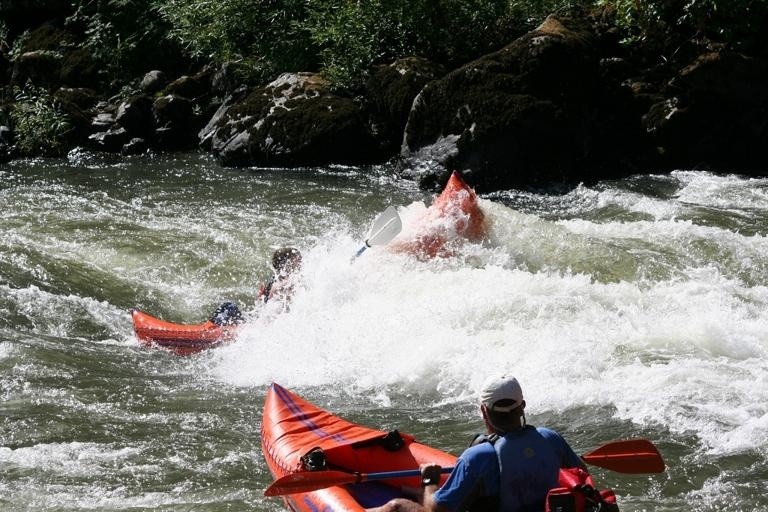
[481,374,523,412]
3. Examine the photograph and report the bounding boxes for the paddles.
[263,437,664,497]
[349,205,401,263]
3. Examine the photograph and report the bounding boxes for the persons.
[365,374,588,512]
[255,248,302,304]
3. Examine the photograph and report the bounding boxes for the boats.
[130,169,488,361]
[261,381,618,512]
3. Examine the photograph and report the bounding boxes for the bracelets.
[421,477,440,486]
